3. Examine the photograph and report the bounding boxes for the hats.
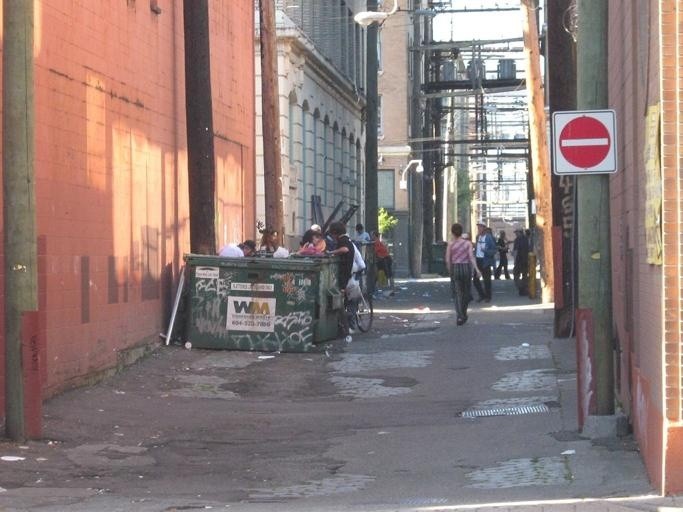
[513,227,523,233]
[311,224,321,233]
[477,222,487,227]
[244,240,256,249]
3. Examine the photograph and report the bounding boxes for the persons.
[215,238,255,259]
[296,222,395,336]
[258,226,279,256]
[459,220,532,303]
[443,223,482,327]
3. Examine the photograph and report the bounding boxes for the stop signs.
[552,109,618,175]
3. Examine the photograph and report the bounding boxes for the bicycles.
[346,272,374,333]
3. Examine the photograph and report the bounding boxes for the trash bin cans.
[186,254,344,353]
[431,241,449,275]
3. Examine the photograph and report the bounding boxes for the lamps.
[353,0,398,27]
[399,158,424,188]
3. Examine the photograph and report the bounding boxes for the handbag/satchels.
[351,244,366,274]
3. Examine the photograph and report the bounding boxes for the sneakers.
[457,316,468,325]
[476,295,491,303]
